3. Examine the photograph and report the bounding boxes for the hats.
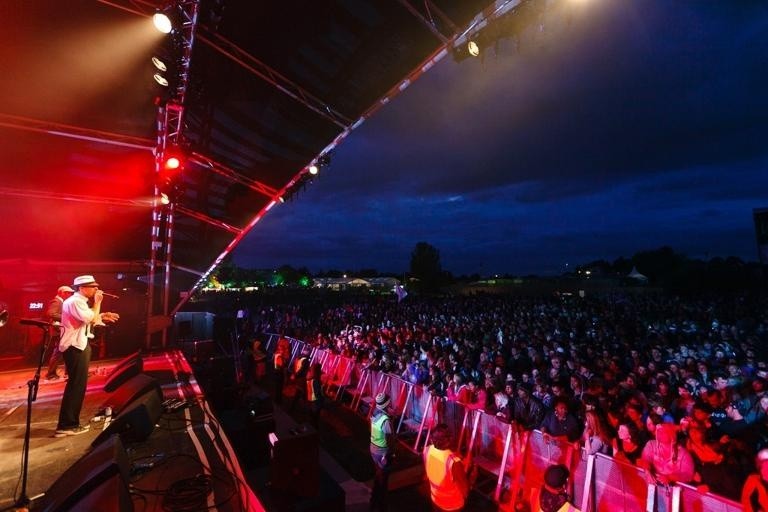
[58,275,99,292]
[376,393,392,410]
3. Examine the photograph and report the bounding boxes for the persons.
[44,286,75,380]
[200,286,768,511]
[54,275,120,439]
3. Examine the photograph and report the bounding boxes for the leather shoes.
[54,423,90,438]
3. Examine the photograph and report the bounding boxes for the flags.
[394,281,406,301]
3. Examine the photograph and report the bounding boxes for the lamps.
[279,150,335,203]
[453,40,479,64]
[151,5,181,206]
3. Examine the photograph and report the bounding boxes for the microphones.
[20,318,61,331]
[104,292,120,302]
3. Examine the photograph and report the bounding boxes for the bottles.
[89,406,112,422]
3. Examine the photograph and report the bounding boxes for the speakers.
[103,362,139,390]
[105,351,143,378]
[92,391,161,447]
[44,437,129,512]
[101,374,164,414]
